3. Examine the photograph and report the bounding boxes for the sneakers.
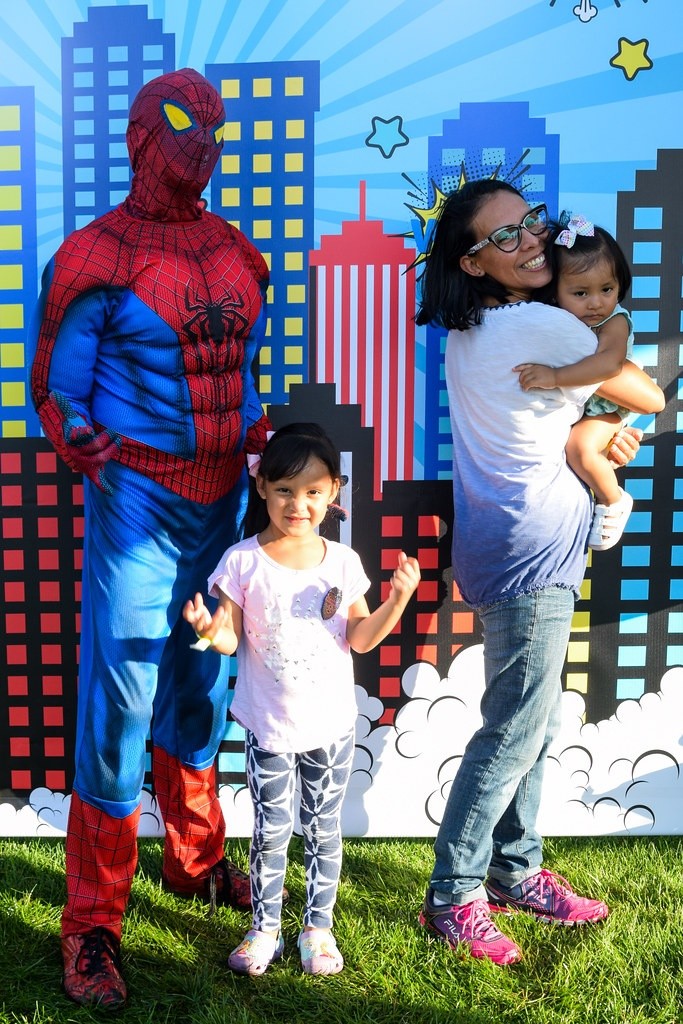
[61,927,128,1012]
[162,856,289,911]
[484,869,608,926]
[418,898,522,965]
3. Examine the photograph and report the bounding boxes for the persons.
[415,179,666,972]
[28,68,348,1009]
[180,421,421,979]
[512,217,637,551]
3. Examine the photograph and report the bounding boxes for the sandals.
[588,486,633,551]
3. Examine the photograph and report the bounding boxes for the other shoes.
[228,929,284,974]
[297,927,344,976]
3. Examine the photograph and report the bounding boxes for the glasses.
[466,204,548,256]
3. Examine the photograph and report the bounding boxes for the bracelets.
[190,630,220,652]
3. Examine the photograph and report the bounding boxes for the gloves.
[49,391,124,496]
